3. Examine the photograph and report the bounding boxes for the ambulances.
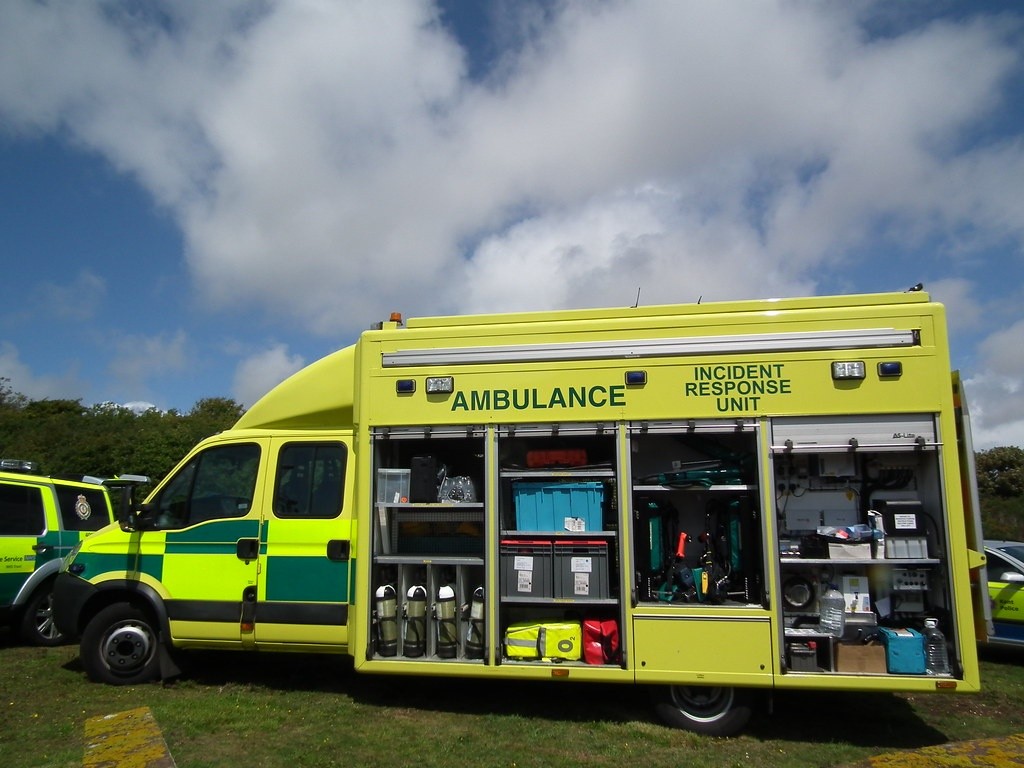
[53,280,989,740]
[0,456,155,645]
[977,537,1024,647]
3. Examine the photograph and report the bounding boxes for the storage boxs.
[500,540,553,598]
[835,642,887,673]
[513,481,605,531]
[554,540,610,599]
[878,626,926,674]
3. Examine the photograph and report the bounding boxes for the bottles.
[818,583,845,638]
[920,618,952,677]
